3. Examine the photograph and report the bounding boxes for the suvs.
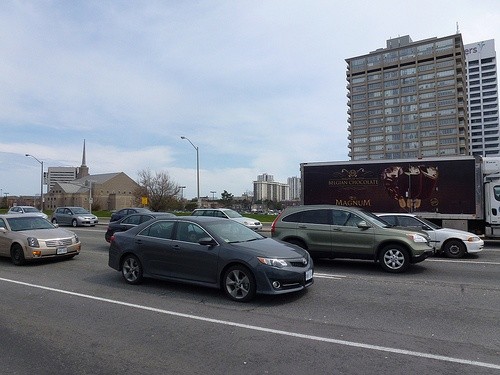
[271,205,435,273]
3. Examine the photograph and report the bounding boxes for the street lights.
[180,136,200,209]
[4,193,9,206]
[179,186,186,199]
[210,191,216,206]
[25,154,44,212]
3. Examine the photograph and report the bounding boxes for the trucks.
[300,154,500,241]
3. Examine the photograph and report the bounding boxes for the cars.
[50,206,99,228]
[189,208,263,232]
[105,212,179,243]
[0,214,82,266]
[343,211,485,258]
[111,207,154,222]
[7,206,49,221]
[108,216,314,303]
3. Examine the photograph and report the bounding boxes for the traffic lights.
[43,172,47,178]
